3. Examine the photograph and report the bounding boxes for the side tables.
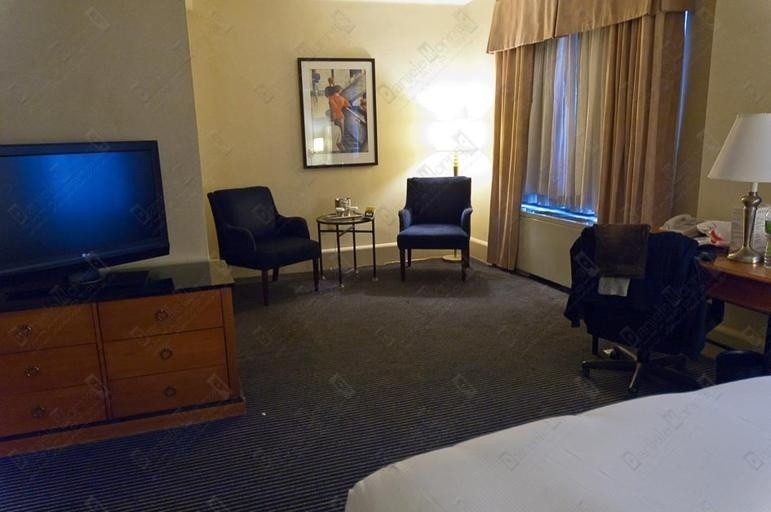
[316,210,377,287]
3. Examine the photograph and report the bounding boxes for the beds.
[344,375,771,511]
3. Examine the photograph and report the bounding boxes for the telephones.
[659,213,708,238]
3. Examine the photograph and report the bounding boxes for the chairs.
[562,223,727,394]
[397,176,473,282]
[207,185,321,304]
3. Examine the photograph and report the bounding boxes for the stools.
[0,266,108,462]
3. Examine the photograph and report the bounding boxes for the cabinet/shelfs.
[93,259,245,439]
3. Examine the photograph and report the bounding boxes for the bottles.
[762,208,770,267]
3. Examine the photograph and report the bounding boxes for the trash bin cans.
[715,349,769,384]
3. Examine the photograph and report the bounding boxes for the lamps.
[707,111,771,265]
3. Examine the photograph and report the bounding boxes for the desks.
[699,251,771,355]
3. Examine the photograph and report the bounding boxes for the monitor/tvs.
[0,139,170,286]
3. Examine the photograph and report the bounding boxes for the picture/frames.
[297,56,378,169]
